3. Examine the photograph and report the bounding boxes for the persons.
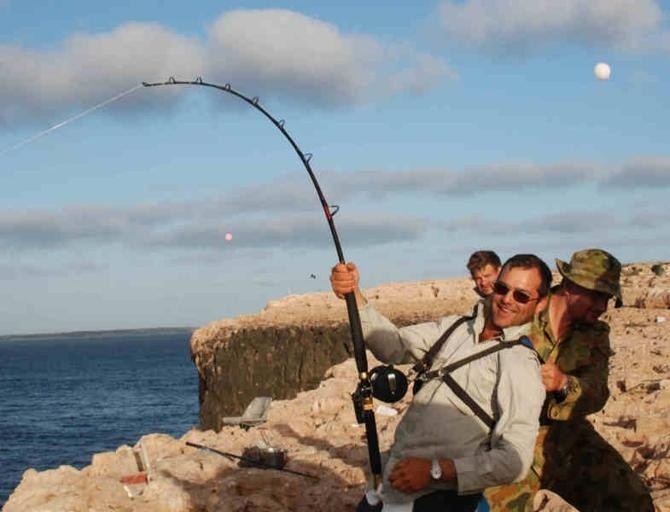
[474,247,657,512]
[466,251,504,319]
[327,252,548,512]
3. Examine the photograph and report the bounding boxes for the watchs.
[428,457,444,481]
[558,376,572,396]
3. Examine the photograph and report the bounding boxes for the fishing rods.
[186,442,320,480]
[0,80,384,487]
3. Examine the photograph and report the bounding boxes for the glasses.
[492,279,539,304]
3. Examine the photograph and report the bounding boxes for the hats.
[556,249,623,308]
